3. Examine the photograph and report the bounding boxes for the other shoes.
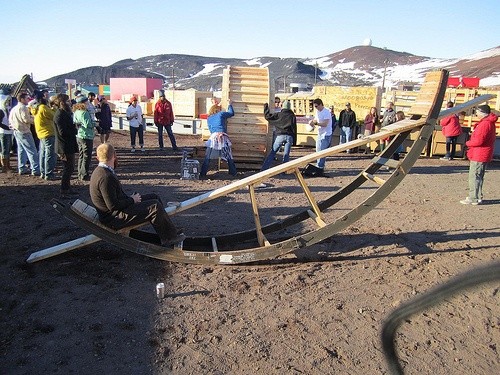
[162,235,185,246]
[41,173,62,180]
[60,191,79,198]
[131,148,136,152]
[19,169,29,174]
[441,156,453,161]
[173,146,181,150]
[160,146,164,150]
[79,176,90,180]
[174,227,185,234]
[140,148,145,151]
[32,172,40,175]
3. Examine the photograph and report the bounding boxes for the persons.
[154,91,180,151]
[439,101,471,161]
[199,98,239,179]
[308,98,333,172]
[338,102,356,154]
[0,88,113,183]
[52,94,82,200]
[362,102,406,161]
[328,104,337,148]
[460,105,499,205]
[126,96,146,153]
[90,143,186,246]
[260,97,297,171]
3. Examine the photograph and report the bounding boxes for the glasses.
[274,100,280,104]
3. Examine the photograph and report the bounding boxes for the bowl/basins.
[199,114,208,119]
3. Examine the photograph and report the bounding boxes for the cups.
[156,283,164,298]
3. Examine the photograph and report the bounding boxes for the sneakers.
[460,197,482,206]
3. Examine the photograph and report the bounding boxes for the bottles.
[135,110,138,119]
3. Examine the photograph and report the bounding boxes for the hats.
[129,97,137,104]
[159,90,165,97]
[345,102,350,106]
[477,104,491,113]
[76,93,87,103]
[281,100,290,110]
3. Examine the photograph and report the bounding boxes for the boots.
[1,154,14,172]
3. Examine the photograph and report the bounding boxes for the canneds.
[156,283,165,299]
